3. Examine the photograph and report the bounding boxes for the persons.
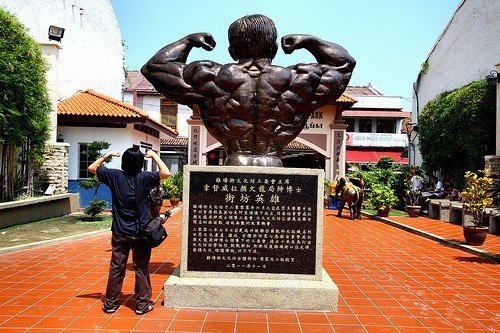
[411,171,462,214]
[87,150,171,315]
[140,14,357,167]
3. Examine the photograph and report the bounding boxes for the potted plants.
[370,182,399,217]
[459,164,493,246]
[161,170,184,207]
[402,164,427,218]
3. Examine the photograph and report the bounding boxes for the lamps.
[47,25,66,43]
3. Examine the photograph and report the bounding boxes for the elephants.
[336,177,362,220]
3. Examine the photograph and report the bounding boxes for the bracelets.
[101,156,106,159]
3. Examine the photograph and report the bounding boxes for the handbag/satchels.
[139,218,167,248]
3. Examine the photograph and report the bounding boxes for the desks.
[328,195,342,209]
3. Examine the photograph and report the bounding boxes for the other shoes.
[136,303,153,313]
[103,304,115,312]
